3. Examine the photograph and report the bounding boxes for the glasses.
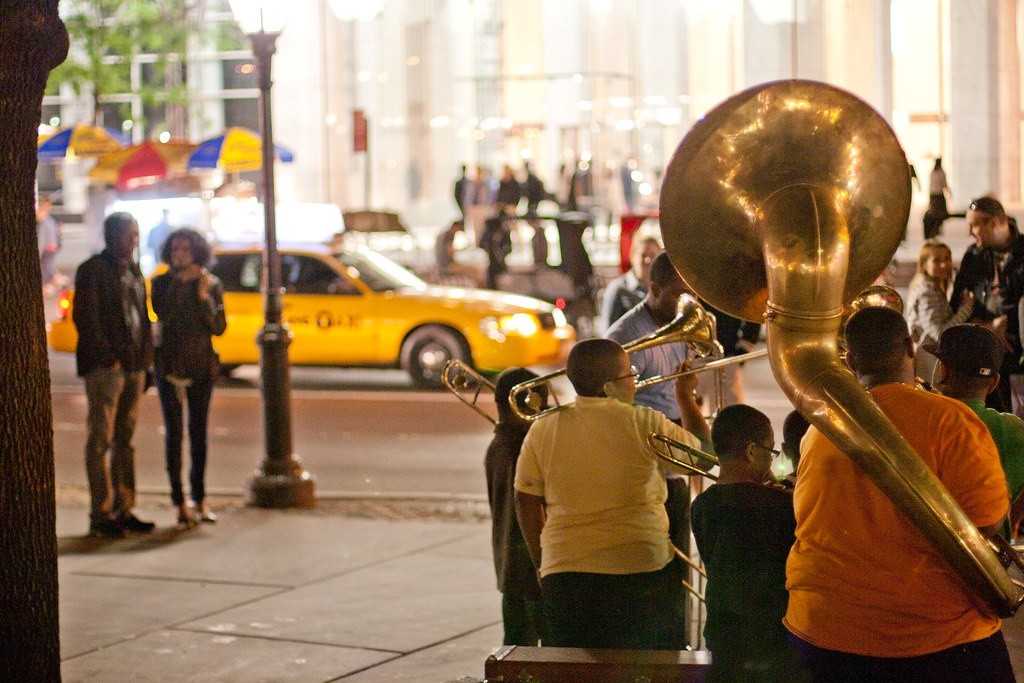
[608,363,641,384]
[754,445,781,457]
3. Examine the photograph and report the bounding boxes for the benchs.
[485,645,711,683]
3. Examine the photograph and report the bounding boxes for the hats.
[923,325,1005,380]
[493,367,553,411]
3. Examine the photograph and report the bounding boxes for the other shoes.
[115,511,155,533]
[178,504,218,531]
[734,339,755,353]
[89,515,129,539]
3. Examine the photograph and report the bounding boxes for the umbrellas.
[37,121,294,186]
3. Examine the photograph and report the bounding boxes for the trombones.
[646,432,1024,604]
[441,360,708,602]
[852,284,904,314]
[682,312,724,650]
[508,293,769,422]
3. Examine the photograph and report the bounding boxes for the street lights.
[227,0,316,505]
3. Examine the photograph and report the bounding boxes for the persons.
[151,228,226,527]
[688,402,796,683]
[781,306,1014,683]
[34,195,64,285]
[147,207,178,265]
[601,233,811,494]
[71,212,156,538]
[430,160,595,289]
[910,159,1024,419]
[486,366,547,646]
[513,338,715,652]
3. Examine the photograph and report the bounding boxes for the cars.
[48,198,575,390]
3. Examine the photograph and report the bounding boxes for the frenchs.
[658,78,1024,619]
